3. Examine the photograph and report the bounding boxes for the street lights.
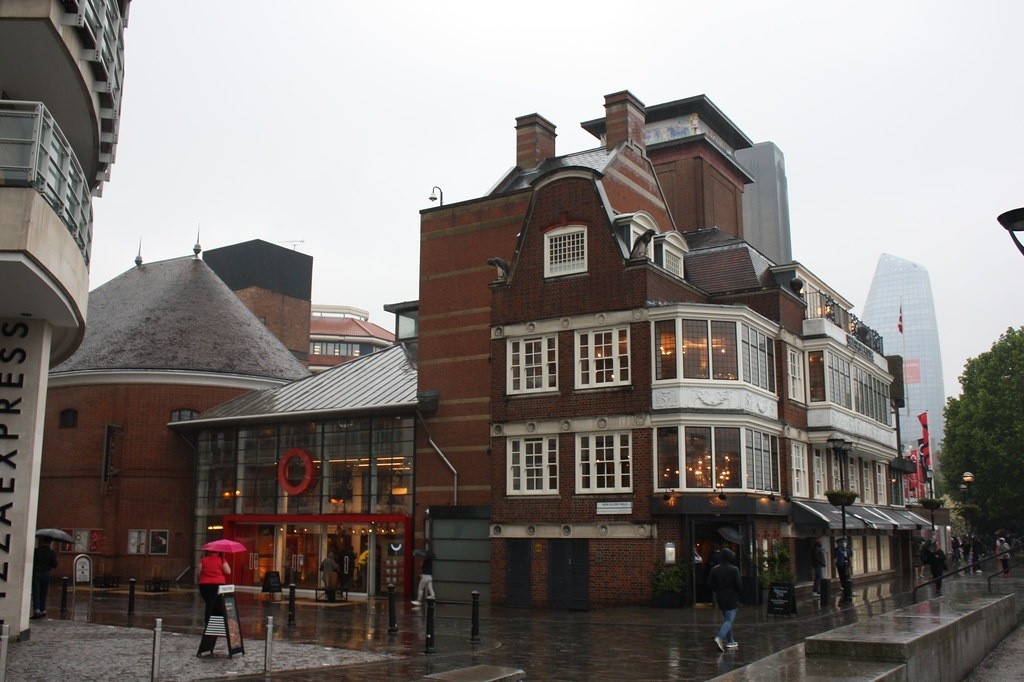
[824,431,852,601]
[963,472,976,533]
[926,466,935,541]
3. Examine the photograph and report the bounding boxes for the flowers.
[824,489,860,498]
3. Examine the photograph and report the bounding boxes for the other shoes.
[411,600,421,606]
[41,612,48,617]
[714,637,724,652]
[726,641,739,648]
[30,613,41,620]
[812,592,820,597]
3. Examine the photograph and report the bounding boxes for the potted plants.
[916,497,946,510]
[649,559,686,609]
[754,543,795,602]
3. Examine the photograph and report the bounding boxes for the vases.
[826,494,855,505]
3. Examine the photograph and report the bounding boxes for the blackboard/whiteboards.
[767,583,798,615]
[198,594,244,655]
[262,571,282,592]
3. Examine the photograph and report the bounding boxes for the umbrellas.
[412,549,436,559]
[36,529,76,544]
[908,536,928,542]
[201,539,246,553]
[718,526,744,544]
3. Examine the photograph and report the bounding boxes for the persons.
[950,535,986,573]
[931,542,945,593]
[991,534,1024,576]
[709,549,742,652]
[30,538,57,619]
[319,554,340,602]
[833,538,854,588]
[708,544,721,606]
[913,542,925,578]
[197,552,231,632]
[412,558,435,606]
[812,537,827,596]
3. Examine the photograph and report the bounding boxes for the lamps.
[663,486,674,501]
[755,486,775,501]
[780,488,791,503]
[714,484,727,501]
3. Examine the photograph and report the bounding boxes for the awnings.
[794,500,939,530]
